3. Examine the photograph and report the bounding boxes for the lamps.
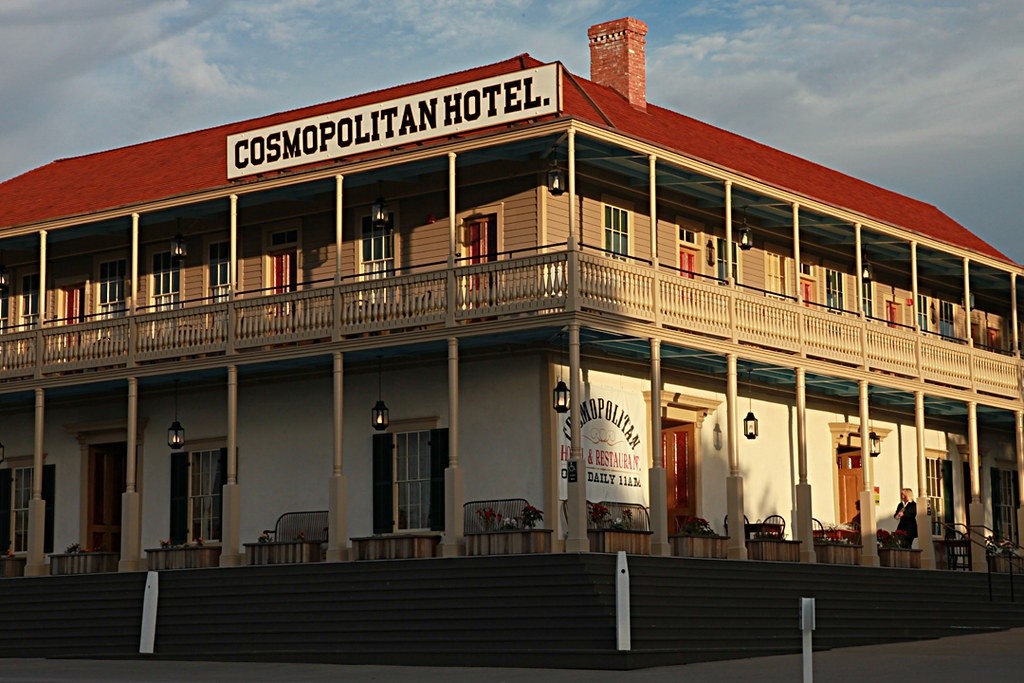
[552,332,570,413]
[967,452,983,470]
[736,205,753,251]
[372,179,389,227]
[868,391,881,457]
[167,378,185,449]
[0,442,4,464]
[862,243,873,283]
[170,216,187,260]
[0,248,10,290]
[372,354,390,430]
[547,144,565,195]
[744,369,758,439]
[961,273,974,311]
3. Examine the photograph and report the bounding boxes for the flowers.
[679,516,717,537]
[476,505,543,527]
[986,536,1015,555]
[876,528,907,549]
[159,536,205,548]
[259,531,306,543]
[7,545,17,558]
[590,504,632,531]
[65,542,101,553]
[813,528,860,544]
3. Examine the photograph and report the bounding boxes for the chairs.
[844,522,861,545]
[562,499,595,553]
[756,514,786,543]
[724,514,749,536]
[811,517,824,540]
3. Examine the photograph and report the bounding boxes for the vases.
[464,529,554,556]
[145,545,222,571]
[0,556,26,578]
[991,556,1024,572]
[242,542,323,564]
[668,535,732,558]
[587,530,654,554]
[879,549,922,568]
[814,543,864,564]
[48,552,120,575]
[744,540,803,561]
[349,534,441,561]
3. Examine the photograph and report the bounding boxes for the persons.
[894,488,917,548]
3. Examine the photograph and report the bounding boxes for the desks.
[933,539,972,572]
[743,523,767,542]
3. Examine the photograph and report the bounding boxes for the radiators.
[755,527,785,541]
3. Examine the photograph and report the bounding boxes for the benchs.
[599,501,650,531]
[463,498,530,531]
[263,510,329,543]
